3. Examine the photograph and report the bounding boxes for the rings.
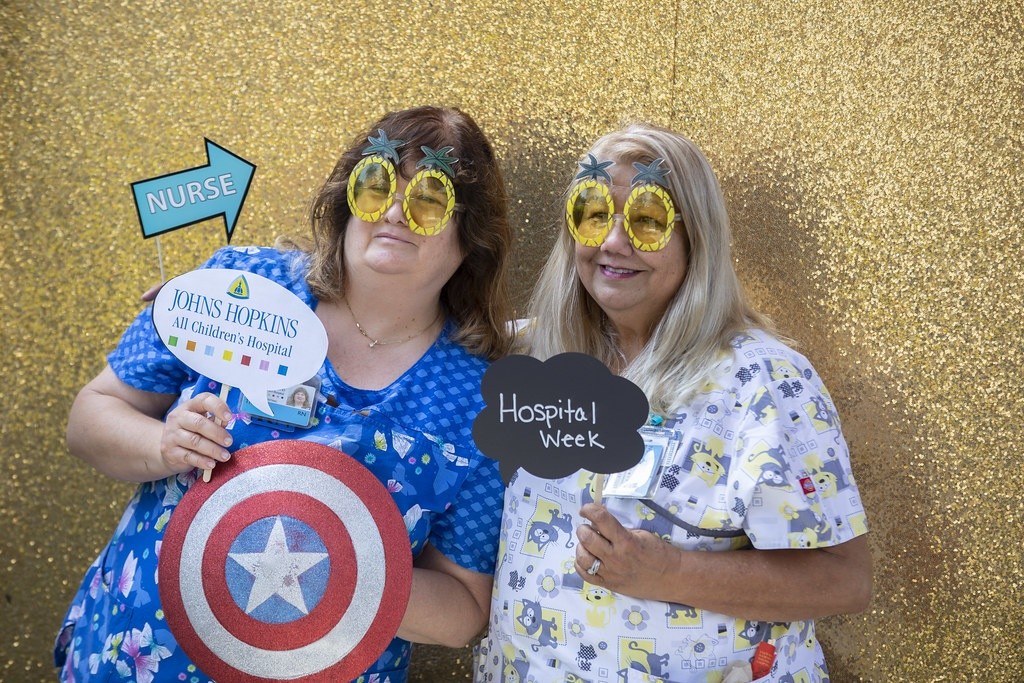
[587,560,600,574]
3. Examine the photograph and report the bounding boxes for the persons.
[52,107,512,683]
[287,388,311,410]
[471,123,872,683]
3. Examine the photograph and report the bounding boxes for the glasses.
[565,153,682,252]
[347,128,468,237]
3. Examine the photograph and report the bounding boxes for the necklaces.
[343,292,443,348]
